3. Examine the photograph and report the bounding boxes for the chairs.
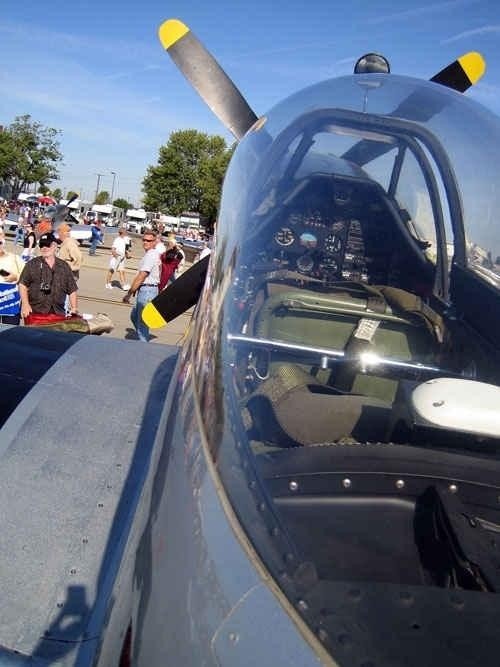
[247,280,442,408]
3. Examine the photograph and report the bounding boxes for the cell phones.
[0,269,10,276]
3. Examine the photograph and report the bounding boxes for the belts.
[142,284,159,288]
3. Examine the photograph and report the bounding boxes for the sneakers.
[105,282,131,290]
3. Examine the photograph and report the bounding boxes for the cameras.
[40,282,51,291]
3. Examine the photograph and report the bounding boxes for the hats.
[39,233,56,244]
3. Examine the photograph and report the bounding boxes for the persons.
[0,192,216,343]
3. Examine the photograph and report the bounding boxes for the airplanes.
[0,16,500,667]
